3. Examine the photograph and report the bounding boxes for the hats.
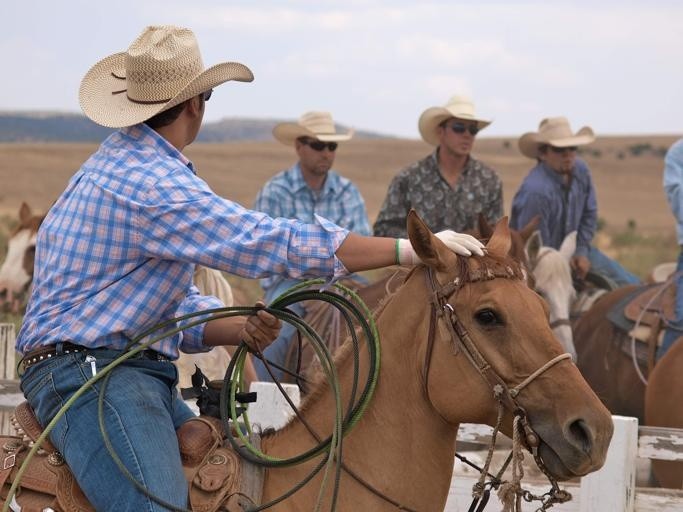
[73,24,255,130]
[519,116,595,159]
[271,109,356,148]
[419,93,491,143]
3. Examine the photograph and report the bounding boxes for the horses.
[0,199,683,512]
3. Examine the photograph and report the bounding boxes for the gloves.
[394,229,487,268]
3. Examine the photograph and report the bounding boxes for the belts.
[23,345,118,361]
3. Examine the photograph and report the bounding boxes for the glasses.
[541,141,580,153]
[299,138,338,151]
[443,123,481,135]
[200,88,214,98]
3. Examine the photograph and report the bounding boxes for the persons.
[253,111,374,383]
[373,97,504,238]
[656,138,683,361]
[15,26,488,512]
[509,116,642,292]
